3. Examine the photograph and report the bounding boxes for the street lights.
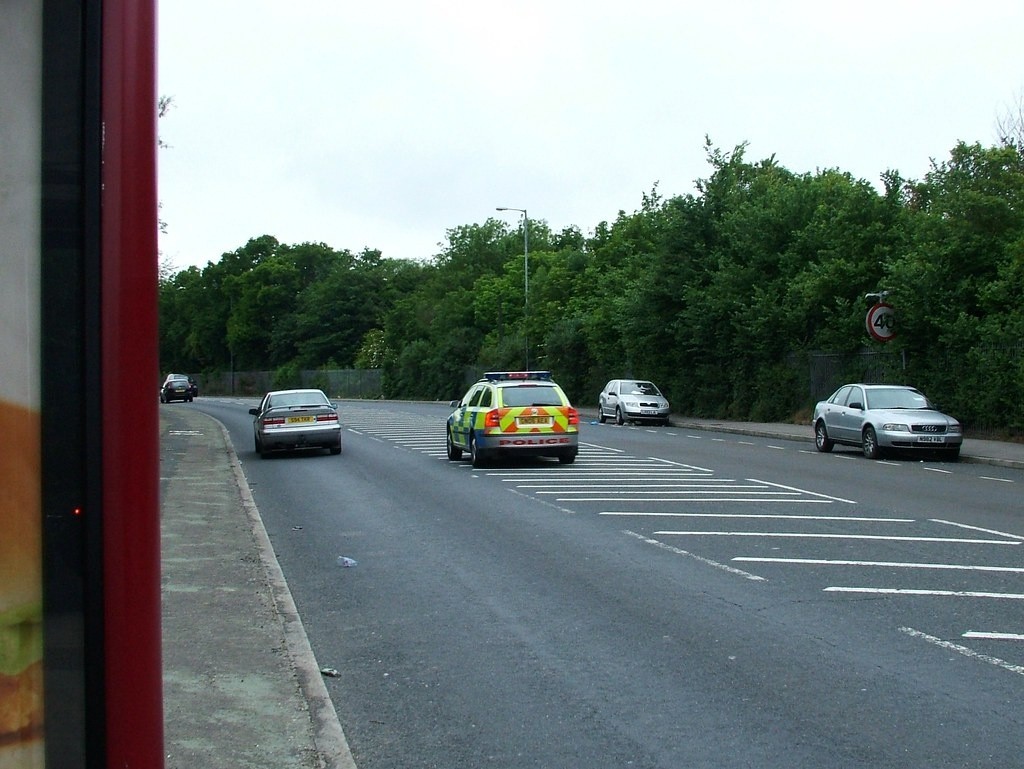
[496,207,529,372]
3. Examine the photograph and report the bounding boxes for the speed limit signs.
[868,303,900,342]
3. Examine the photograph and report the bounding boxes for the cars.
[248,388,342,459]
[167,373,198,397]
[160,378,194,404]
[598,379,670,426]
[445,371,580,469]
[812,383,963,460]
